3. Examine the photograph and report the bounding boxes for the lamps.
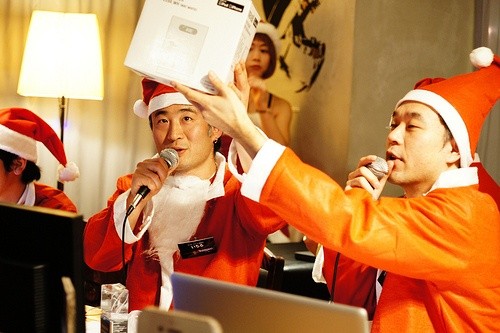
[17,10,104,191]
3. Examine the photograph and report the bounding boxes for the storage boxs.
[123,0,261,96]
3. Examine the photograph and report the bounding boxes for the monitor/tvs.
[169,273,370,333]
[0,200,87,333]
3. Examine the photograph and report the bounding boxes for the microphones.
[366,157,388,187]
[125,149,179,216]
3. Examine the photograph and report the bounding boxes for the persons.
[83,61,289,313]
[0,106,79,213]
[172,47,500,333]
[240,20,293,243]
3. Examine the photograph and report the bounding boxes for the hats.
[255,19,281,60]
[0,107,79,182]
[391,46,500,168]
[133,78,193,119]
